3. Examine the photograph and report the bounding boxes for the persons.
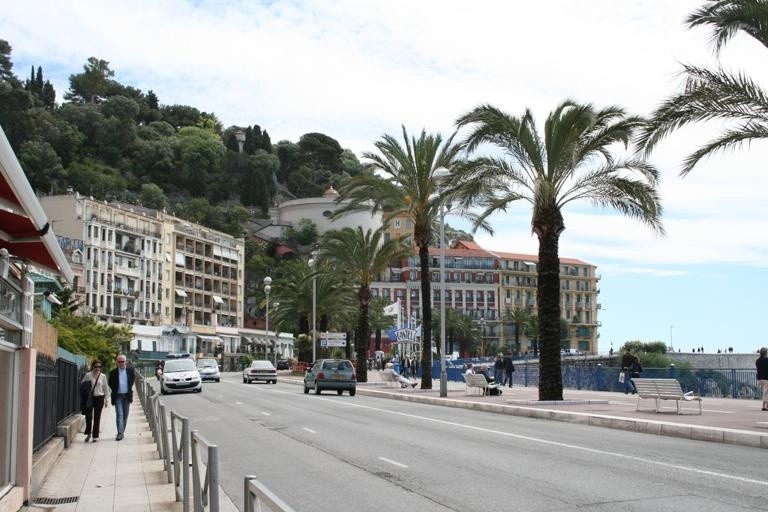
[363,351,419,389]
[108,352,136,440]
[754,348,767,410]
[80,358,107,443]
[691,346,767,352]
[608,347,643,394]
[155,360,162,372]
[467,349,514,395]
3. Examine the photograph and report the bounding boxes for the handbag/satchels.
[79,391,93,409]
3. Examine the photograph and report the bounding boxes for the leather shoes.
[116,435,121,440]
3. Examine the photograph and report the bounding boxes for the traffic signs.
[320,339,346,347]
[321,332,346,339]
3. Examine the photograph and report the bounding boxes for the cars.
[370,348,458,364]
[195,357,222,383]
[302,358,356,397]
[500,344,583,359]
[158,352,202,395]
[241,359,277,385]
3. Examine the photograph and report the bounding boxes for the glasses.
[118,361,124,363]
[93,365,102,368]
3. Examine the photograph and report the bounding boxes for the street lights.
[273,300,279,361]
[424,165,453,399]
[475,316,486,358]
[264,275,272,363]
[668,324,674,348]
[305,250,317,363]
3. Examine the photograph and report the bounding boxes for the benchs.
[630,378,701,416]
[378,371,400,388]
[461,374,501,399]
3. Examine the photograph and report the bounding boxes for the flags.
[382,302,399,315]
